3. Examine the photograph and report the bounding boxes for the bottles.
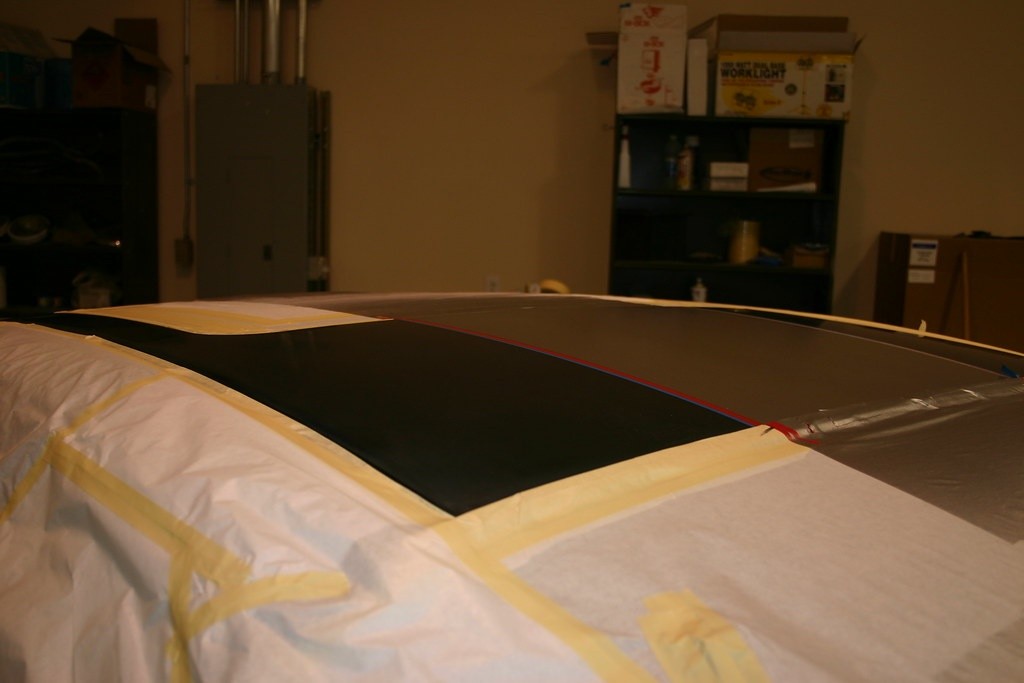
[665,133,703,191]
[617,125,631,188]
[691,277,708,304]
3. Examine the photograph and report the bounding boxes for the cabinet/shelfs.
[0,105,157,318]
[608,112,851,323]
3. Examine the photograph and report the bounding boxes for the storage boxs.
[71,271,111,308]
[586,4,717,112]
[704,161,749,190]
[0,17,172,108]
[715,14,868,118]
[788,243,828,268]
[747,126,823,192]
[688,38,709,114]
[874,230,1024,350]
